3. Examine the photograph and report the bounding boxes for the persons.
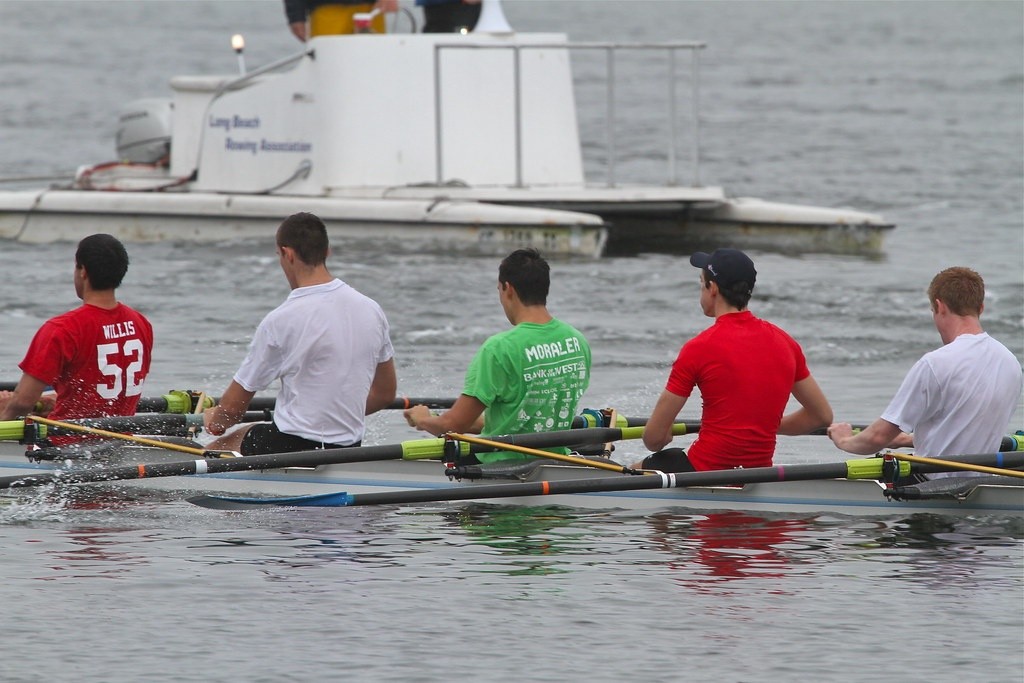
[204,213,397,456]
[0,233,154,460]
[629,248,832,487]
[404,248,591,466]
[827,267,1022,488]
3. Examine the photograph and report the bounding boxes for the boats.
[0,0,899,266]
[2,424,1023,523]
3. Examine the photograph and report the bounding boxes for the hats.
[690,248,757,295]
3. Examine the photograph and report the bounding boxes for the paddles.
[0,420,703,491]
[568,409,870,438]
[183,450,1024,512]
[848,427,1024,455]
[0,409,274,442]
[31,385,460,419]
